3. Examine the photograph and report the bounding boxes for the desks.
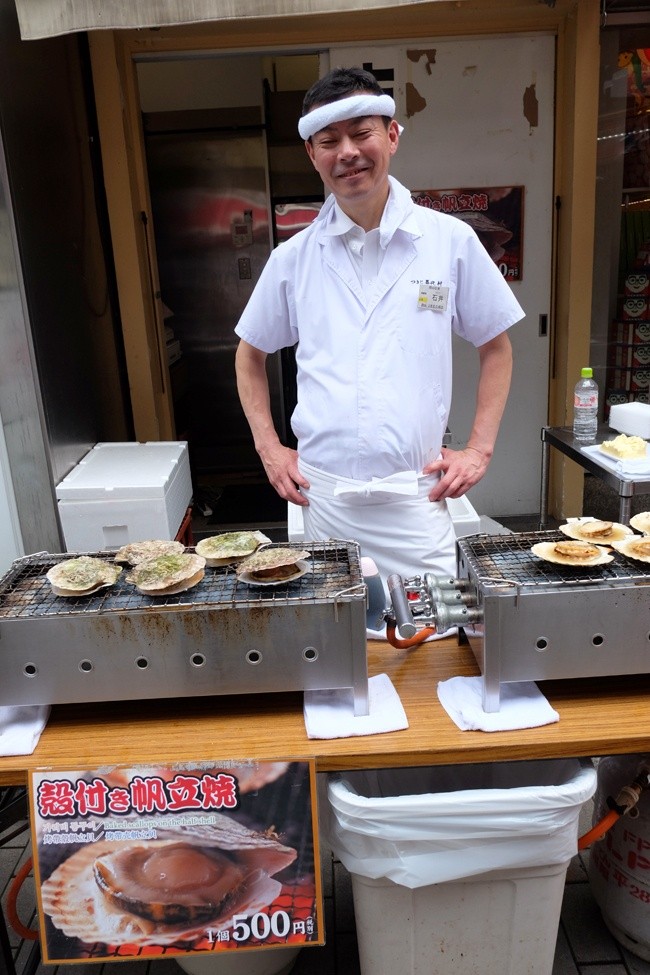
[540,426,650,528]
[0,636,650,786]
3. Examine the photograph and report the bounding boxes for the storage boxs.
[56,441,192,553]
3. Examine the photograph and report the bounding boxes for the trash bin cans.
[324,754,599,975]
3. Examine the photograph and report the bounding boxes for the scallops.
[530,512,650,566]
[46,530,312,598]
[41,811,298,947]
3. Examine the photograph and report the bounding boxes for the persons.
[234,66,525,573]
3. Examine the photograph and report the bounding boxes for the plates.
[597,448,621,462]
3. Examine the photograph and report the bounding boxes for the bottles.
[360,557,386,632]
[574,368,598,440]
